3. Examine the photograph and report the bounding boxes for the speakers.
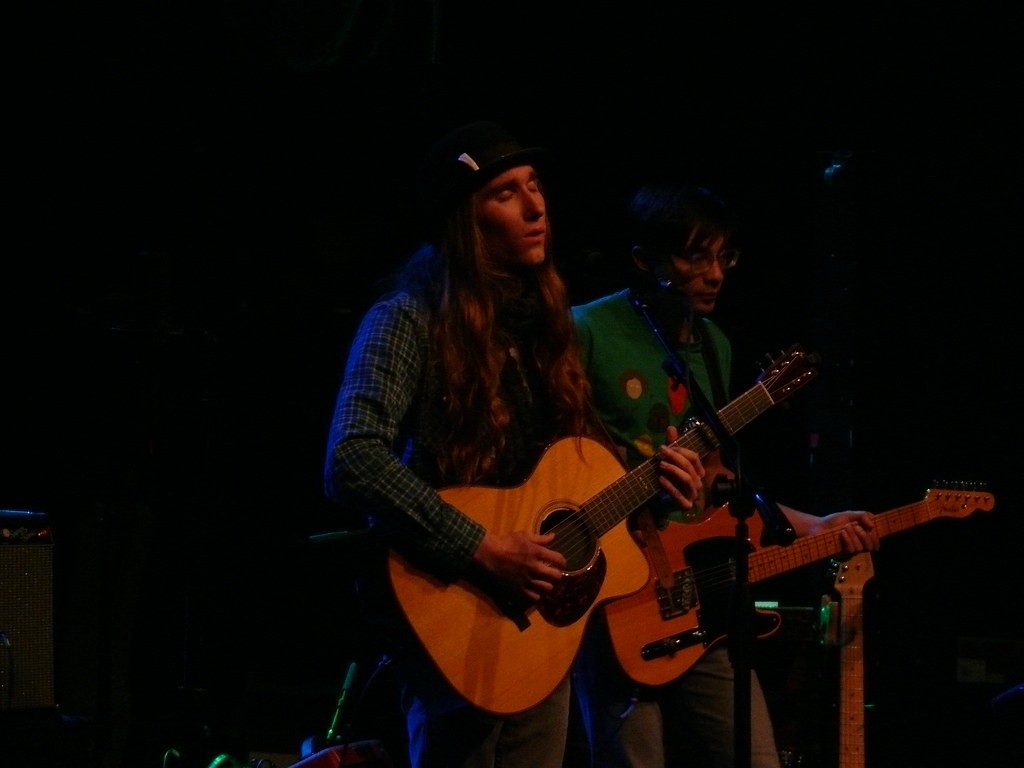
[0,509,54,713]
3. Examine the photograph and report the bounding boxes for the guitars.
[377,338,824,716]
[600,479,996,694]
[828,551,876,768]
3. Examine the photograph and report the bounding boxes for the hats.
[418,121,554,228]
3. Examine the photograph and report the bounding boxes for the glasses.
[670,242,733,266]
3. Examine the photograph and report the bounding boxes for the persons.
[322,135,706,768]
[569,183,881,768]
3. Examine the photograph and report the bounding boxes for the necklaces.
[632,297,688,384]
